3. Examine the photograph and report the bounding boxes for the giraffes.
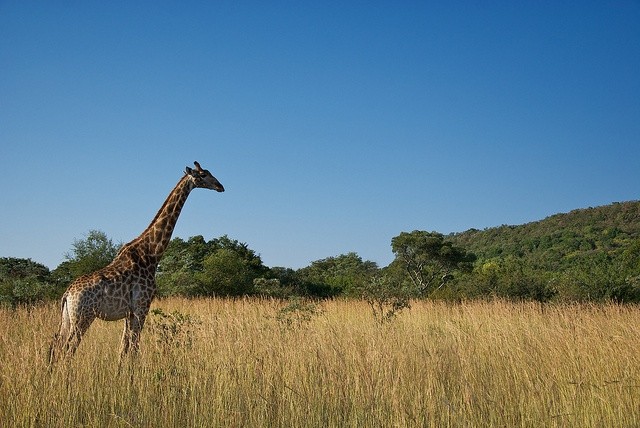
[47,161,225,394]
